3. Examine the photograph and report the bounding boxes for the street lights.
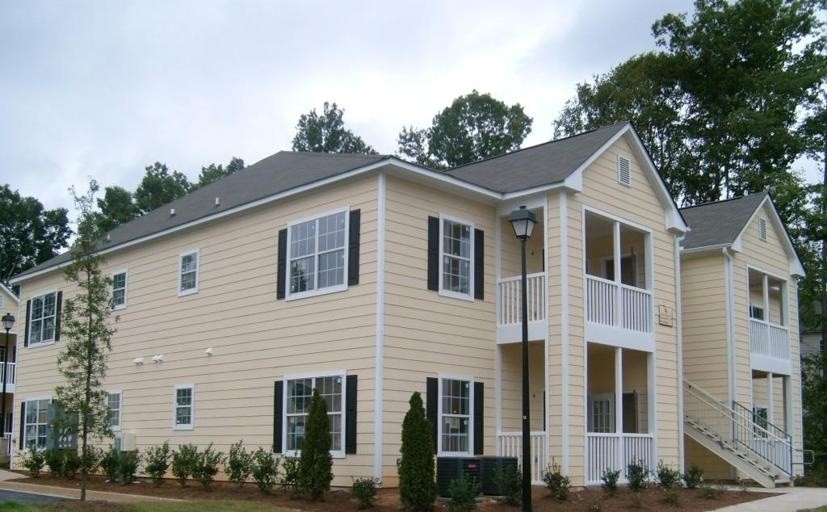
[502,203,542,512]
[0,313,15,440]
